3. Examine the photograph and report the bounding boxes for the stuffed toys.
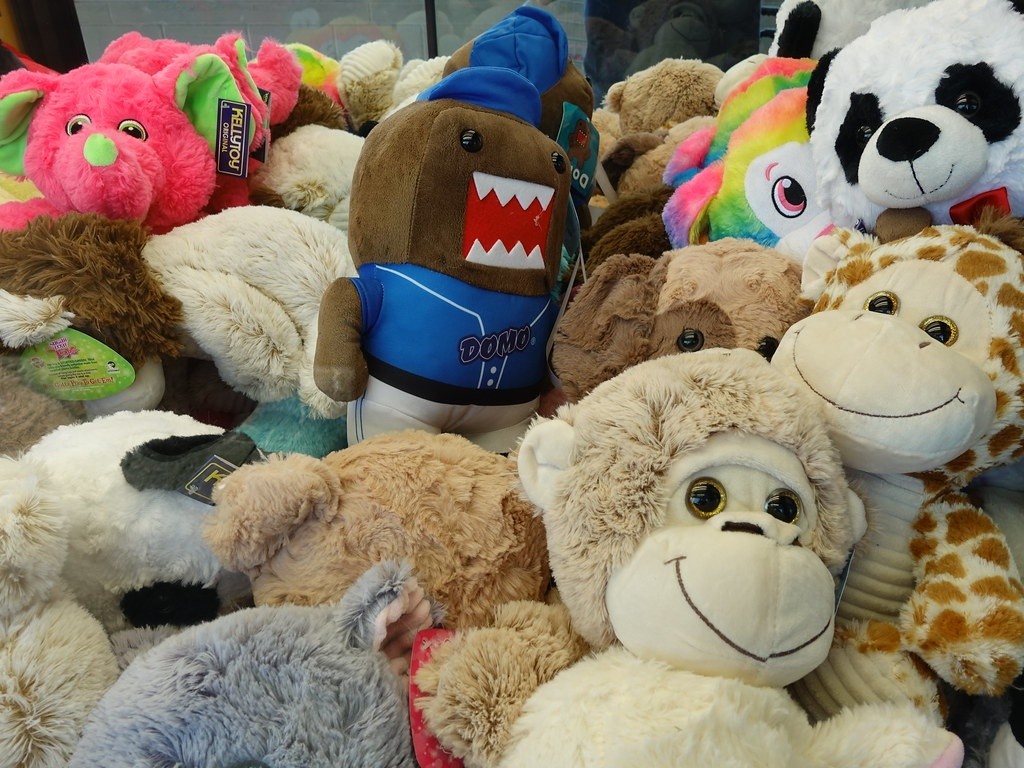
[0,1,1024,767]
